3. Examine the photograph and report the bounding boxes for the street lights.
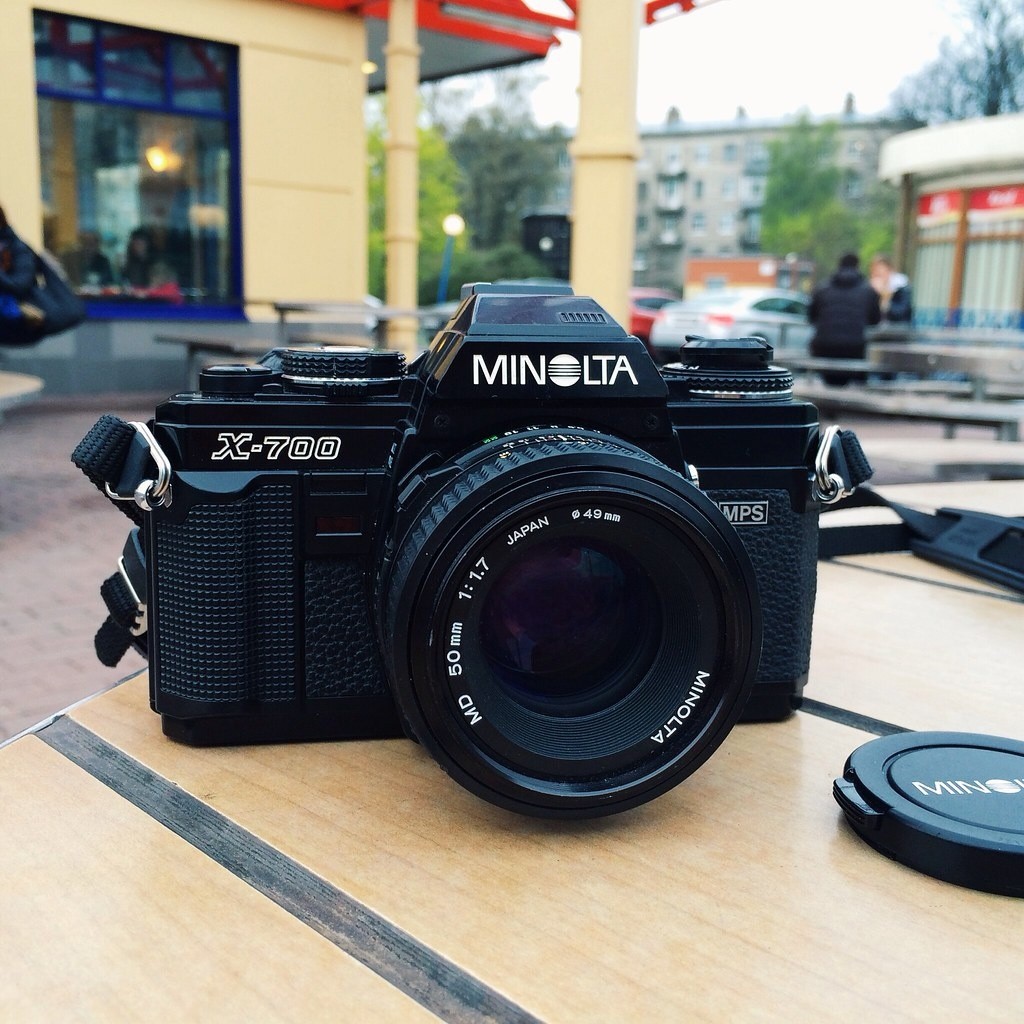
[431,212,469,305]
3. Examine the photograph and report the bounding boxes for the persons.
[807,255,913,388]
[60,225,182,290]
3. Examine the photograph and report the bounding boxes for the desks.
[1,315,1024,1024]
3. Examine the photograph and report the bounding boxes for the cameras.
[138,295,824,822]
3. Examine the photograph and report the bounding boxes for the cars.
[650,287,820,364]
[631,290,680,339]
